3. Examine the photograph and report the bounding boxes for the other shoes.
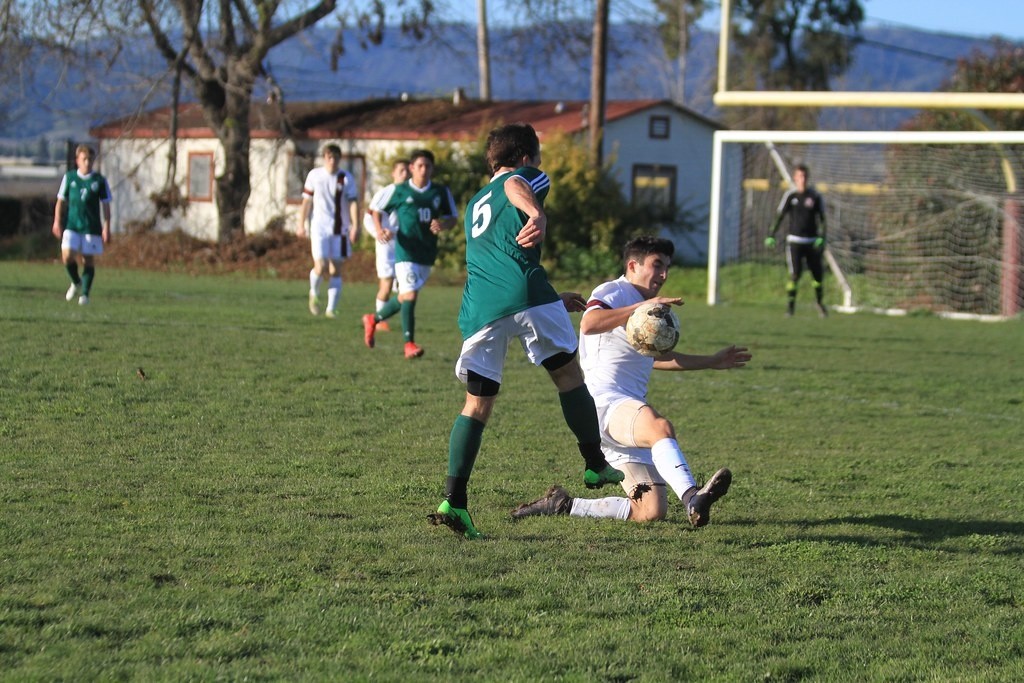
[786,309,794,317]
[817,304,827,318]
[324,310,335,317]
[65,278,82,301]
[309,290,320,315]
[376,320,389,332]
[78,295,88,305]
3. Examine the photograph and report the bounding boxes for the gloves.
[765,237,774,248]
[815,237,824,247]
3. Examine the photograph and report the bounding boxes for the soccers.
[625,303,681,358]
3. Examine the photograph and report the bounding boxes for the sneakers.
[404,341,424,359]
[688,467,732,526]
[512,486,571,518]
[363,314,377,348]
[583,463,624,489]
[437,501,483,539]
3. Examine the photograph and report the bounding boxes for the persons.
[511,236,752,528]
[436,127,625,538]
[296,144,359,318]
[362,150,458,357]
[765,165,828,317]
[362,160,412,331]
[53,146,113,305]
